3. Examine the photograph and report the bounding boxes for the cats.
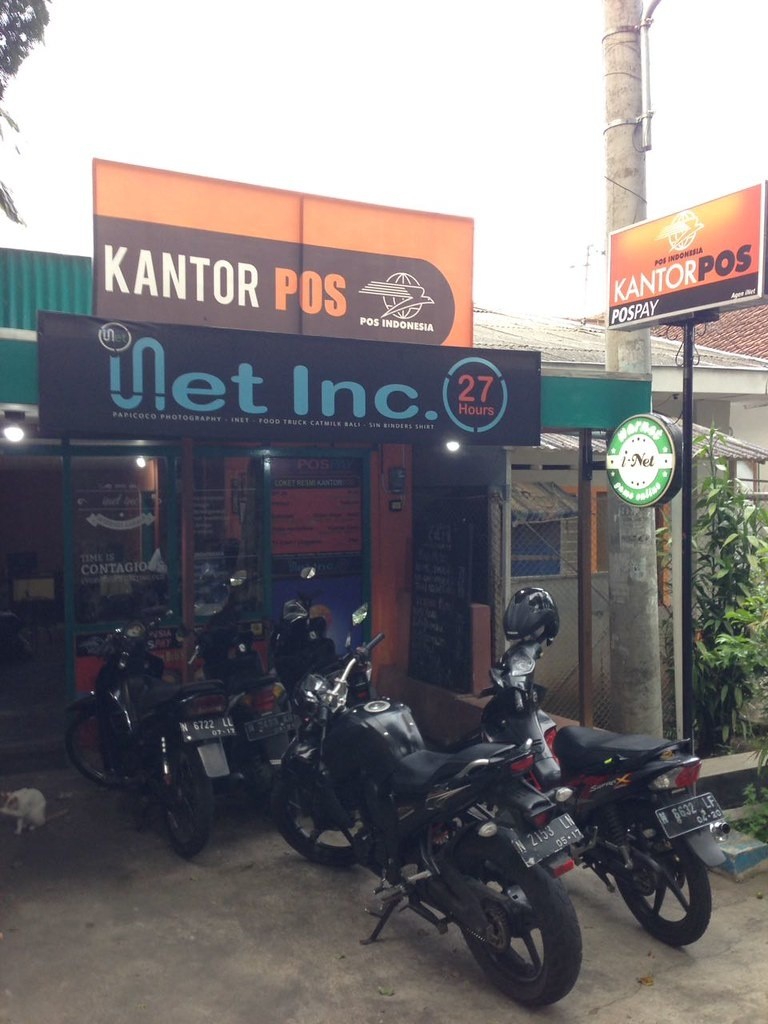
[0,787,70,835]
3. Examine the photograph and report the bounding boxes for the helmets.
[502,586,559,646]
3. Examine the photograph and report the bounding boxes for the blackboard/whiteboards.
[409,484,488,696]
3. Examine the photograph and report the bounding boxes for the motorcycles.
[65,565,373,858]
[450,589,732,950]
[268,600,583,1011]
